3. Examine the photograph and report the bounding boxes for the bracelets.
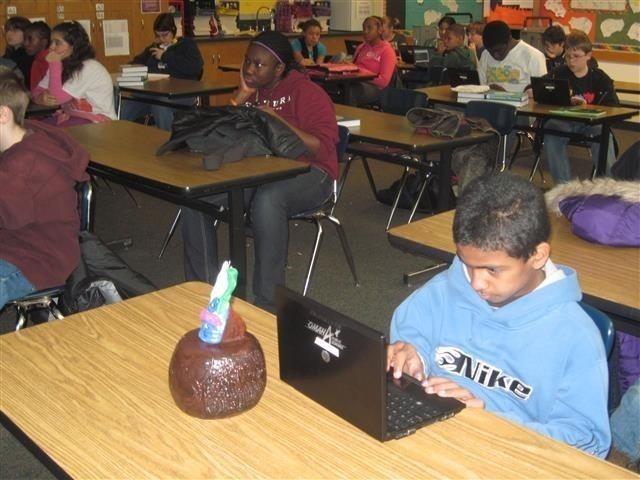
[229,97,238,108]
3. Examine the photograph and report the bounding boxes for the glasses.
[155,32,170,37]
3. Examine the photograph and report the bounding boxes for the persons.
[414,15,488,108]
[449,19,548,181]
[12,20,52,91]
[120,12,204,133]
[30,19,118,122]
[0,64,91,310]
[178,30,340,308]
[341,16,412,110]
[1,16,31,90]
[542,26,598,74]
[384,170,613,461]
[524,29,620,181]
[291,18,327,66]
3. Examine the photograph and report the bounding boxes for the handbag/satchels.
[379,168,454,213]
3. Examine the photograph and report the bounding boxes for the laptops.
[344,37,363,55]
[274,281,466,444]
[447,66,480,87]
[530,76,574,107]
[411,47,431,69]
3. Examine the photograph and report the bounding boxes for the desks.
[0,0,640,480]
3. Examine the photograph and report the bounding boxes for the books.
[116,63,149,88]
[337,115,360,127]
[185,6,240,36]
[148,73,171,82]
